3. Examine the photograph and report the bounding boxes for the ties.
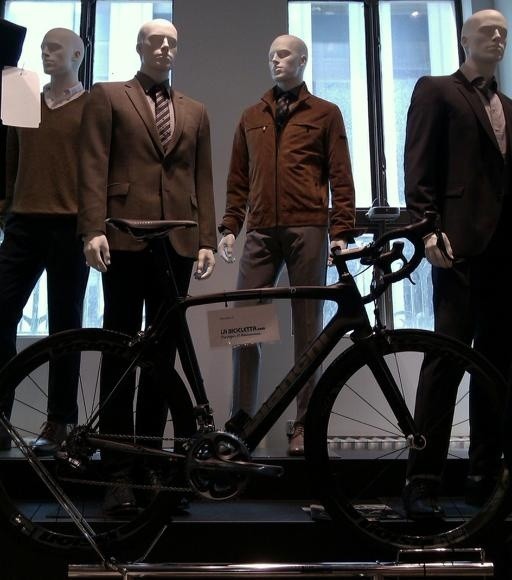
[274,93,290,126]
[152,84,172,155]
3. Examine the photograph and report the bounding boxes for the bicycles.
[1,212,512,565]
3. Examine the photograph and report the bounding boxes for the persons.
[0,26,91,456]
[217,32,357,459]
[402,8,512,520]
[77,17,217,518]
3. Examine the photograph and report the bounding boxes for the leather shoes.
[102,479,144,520]
[32,422,75,457]
[405,481,447,525]
[287,425,307,456]
[143,471,190,511]
